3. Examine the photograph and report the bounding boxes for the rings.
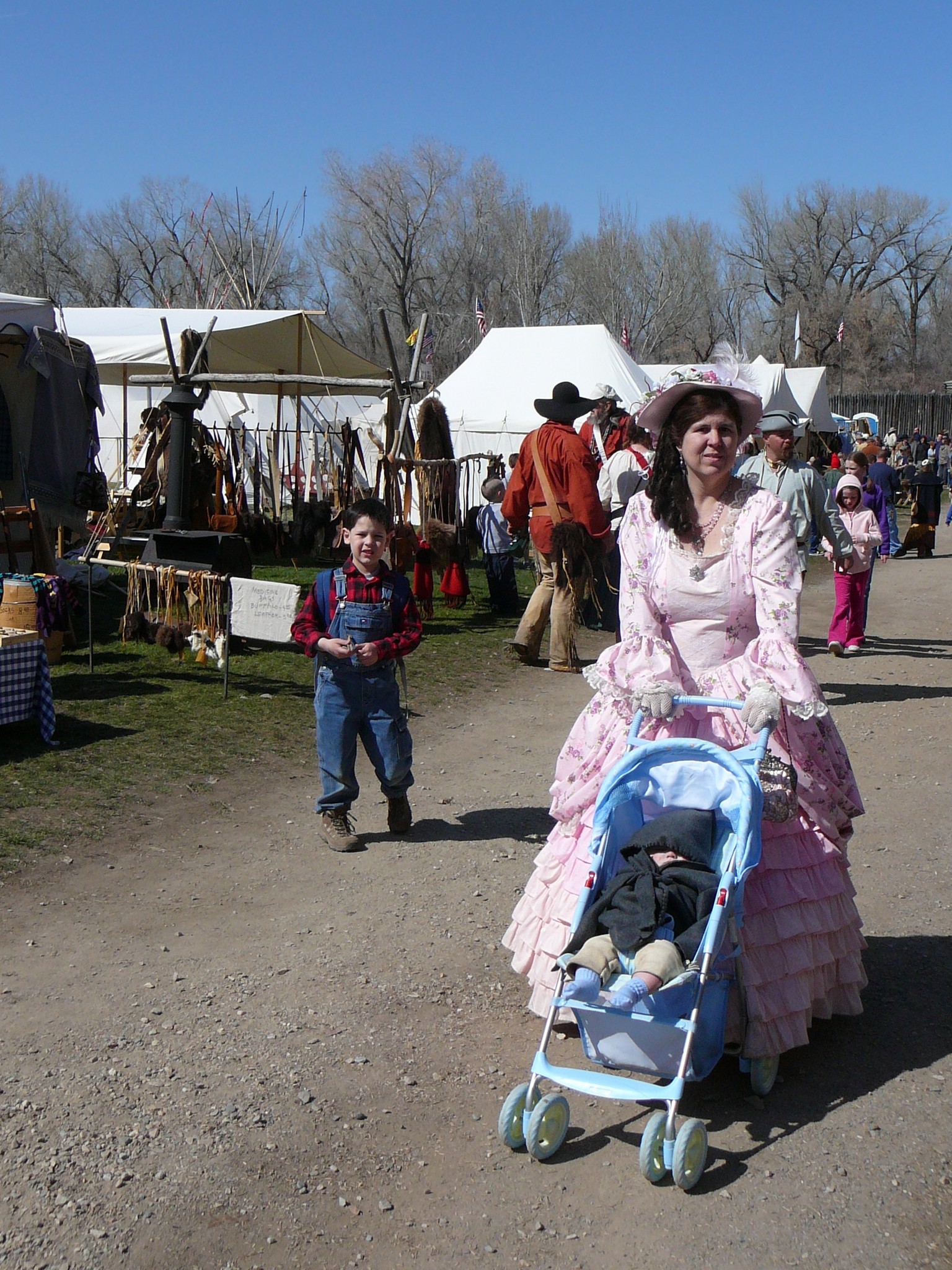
[640,705,649,712]
[762,713,773,722]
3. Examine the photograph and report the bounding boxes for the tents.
[640,362,838,461]
[414,320,658,525]
[55,300,400,500]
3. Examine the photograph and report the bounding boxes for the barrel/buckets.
[0,578,63,665]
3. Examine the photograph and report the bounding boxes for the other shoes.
[513,643,540,665]
[917,550,933,558]
[550,661,583,672]
[891,548,906,558]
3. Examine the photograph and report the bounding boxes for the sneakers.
[808,550,827,556]
[828,641,844,658]
[848,645,859,652]
[386,794,412,836]
[317,809,359,851]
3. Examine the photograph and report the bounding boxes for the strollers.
[494,691,774,1192]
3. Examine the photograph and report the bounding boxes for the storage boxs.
[0,580,64,665]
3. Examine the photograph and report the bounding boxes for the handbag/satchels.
[742,697,799,824]
[117,612,230,668]
[137,475,529,561]
[553,520,607,588]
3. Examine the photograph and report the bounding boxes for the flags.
[621,323,628,352]
[794,309,800,362]
[836,316,845,343]
[475,297,487,339]
[406,325,434,365]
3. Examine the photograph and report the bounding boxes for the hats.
[631,340,763,446]
[943,429,949,434]
[888,427,897,433]
[755,410,799,432]
[588,382,623,402]
[534,381,599,423]
[832,453,841,469]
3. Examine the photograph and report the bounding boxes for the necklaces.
[688,475,733,556]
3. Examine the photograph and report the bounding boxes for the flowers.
[634,367,722,412]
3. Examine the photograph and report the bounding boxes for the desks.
[0,638,61,745]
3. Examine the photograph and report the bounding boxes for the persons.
[500,382,615,674]
[509,454,530,566]
[132,424,148,464]
[141,408,159,432]
[500,367,870,1062]
[580,384,952,631]
[563,808,722,1009]
[290,497,423,852]
[475,476,520,616]
[827,474,884,655]
